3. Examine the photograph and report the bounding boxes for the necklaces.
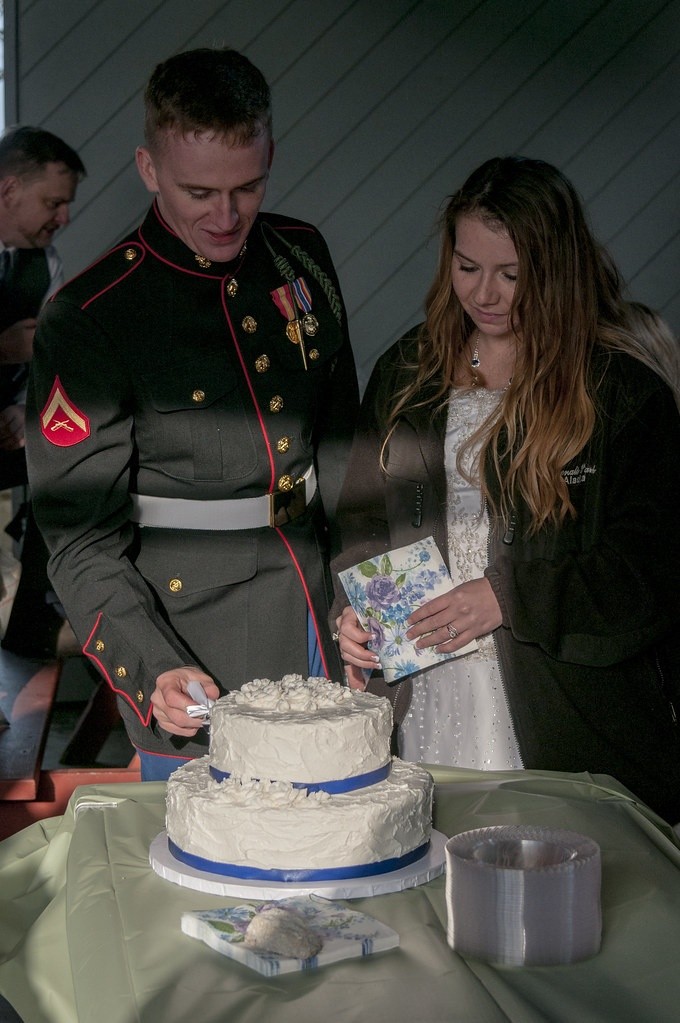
[471,330,513,390]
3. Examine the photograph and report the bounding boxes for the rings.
[447,624,458,638]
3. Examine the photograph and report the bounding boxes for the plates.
[444,823,602,967]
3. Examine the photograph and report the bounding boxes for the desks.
[0,770,680,1023]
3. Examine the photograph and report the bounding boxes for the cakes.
[164,674,435,882]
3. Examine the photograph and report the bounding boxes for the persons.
[23,50,360,782]
[327,156,680,822]
[0,125,87,661]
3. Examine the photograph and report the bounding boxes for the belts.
[130,461,317,531]
